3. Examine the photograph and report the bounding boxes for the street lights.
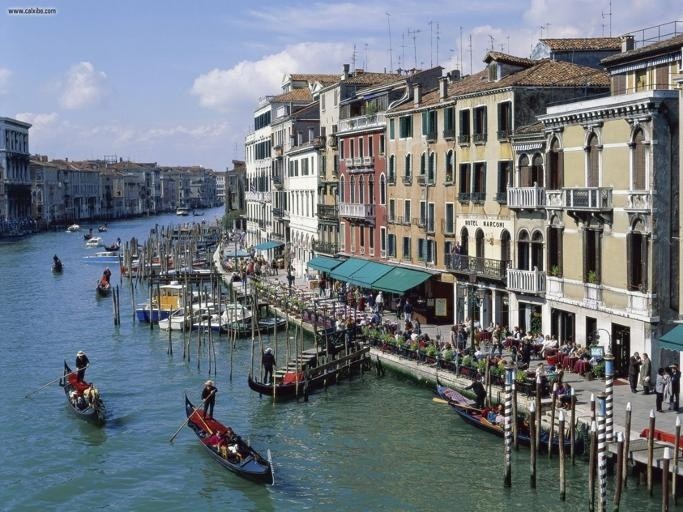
[245,344,370,398]
[457,271,486,369]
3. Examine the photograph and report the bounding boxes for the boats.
[184,392,274,488]
[63,359,106,416]
[67,224,120,251]
[193,212,204,216]
[96,281,109,297]
[435,375,578,452]
[82,251,123,264]
[124,224,286,335]
[50,263,59,273]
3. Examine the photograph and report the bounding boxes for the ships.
[176,208,188,216]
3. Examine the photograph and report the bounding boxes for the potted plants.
[250,281,528,402]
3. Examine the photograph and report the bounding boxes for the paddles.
[433,397,483,412]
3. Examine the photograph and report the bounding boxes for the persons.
[98,267,111,288]
[287,262,293,272]
[76,350,90,385]
[452,241,462,267]
[209,427,250,463]
[290,267,296,287]
[629,352,681,414]
[53,254,58,263]
[263,347,277,384]
[117,236,121,245]
[318,276,594,431]
[68,383,100,411]
[202,379,218,422]
[220,226,278,289]
